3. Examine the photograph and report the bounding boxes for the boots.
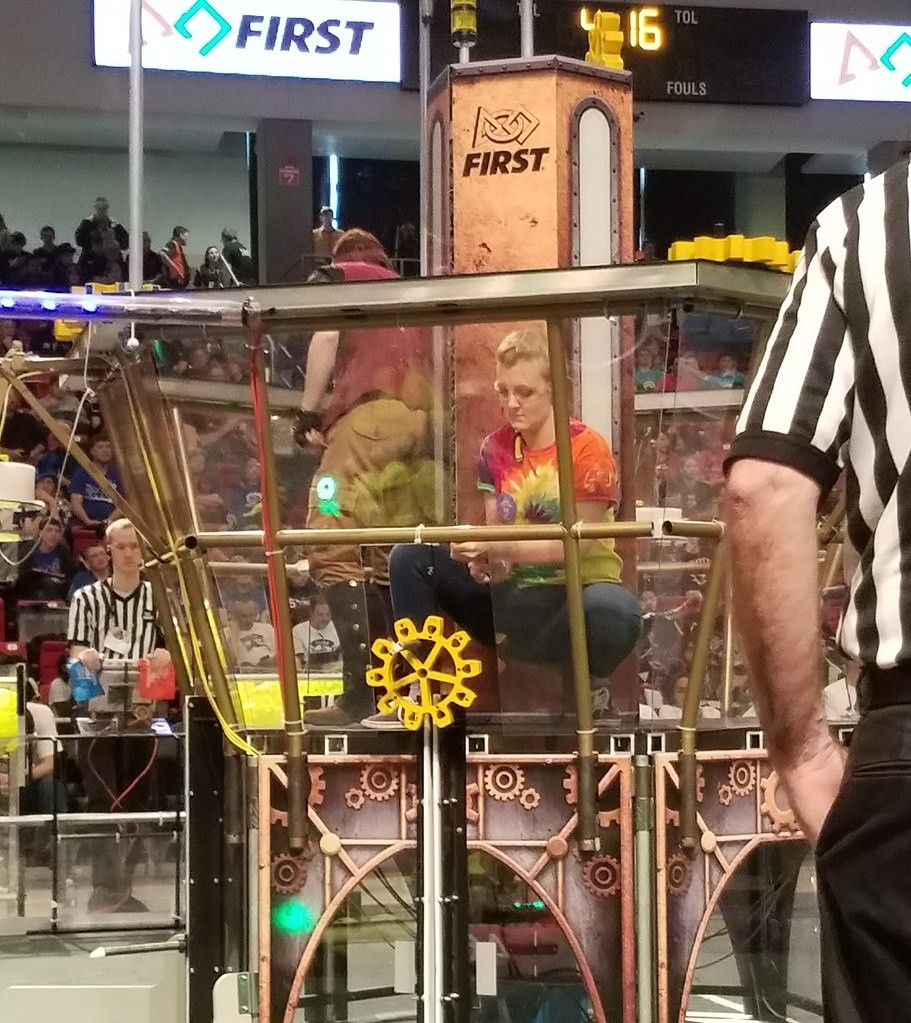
[369,580,405,711]
[301,579,375,725]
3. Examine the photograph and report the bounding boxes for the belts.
[851,660,911,706]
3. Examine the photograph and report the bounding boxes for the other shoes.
[87,888,148,914]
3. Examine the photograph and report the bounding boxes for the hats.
[331,230,392,270]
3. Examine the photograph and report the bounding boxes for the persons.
[2,191,867,873]
[361,325,641,723]
[292,229,431,727]
[730,151,911,1023]
[67,520,187,912]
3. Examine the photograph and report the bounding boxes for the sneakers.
[361,696,431,729]
[591,682,624,726]
